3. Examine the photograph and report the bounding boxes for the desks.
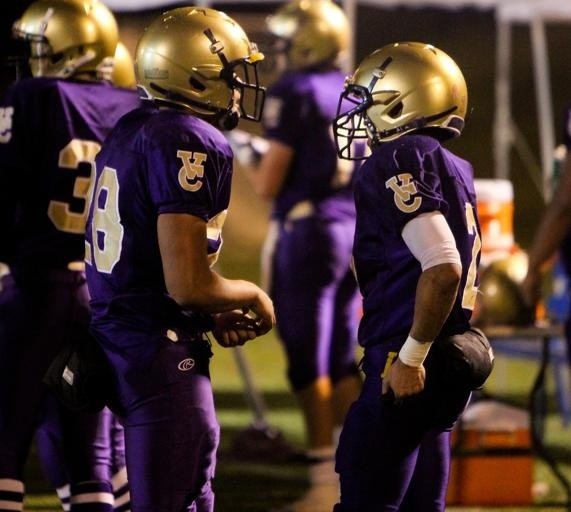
[451,312,571,512]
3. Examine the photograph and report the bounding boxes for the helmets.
[264,0,350,69]
[477,254,538,328]
[331,40,470,162]
[10,0,138,90]
[133,5,267,132]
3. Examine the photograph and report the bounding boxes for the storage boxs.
[444,398,535,506]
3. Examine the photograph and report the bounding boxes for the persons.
[84,5,278,511]
[0,0,147,511]
[332,39,493,512]
[261,0,362,459]
[531,140,570,420]
